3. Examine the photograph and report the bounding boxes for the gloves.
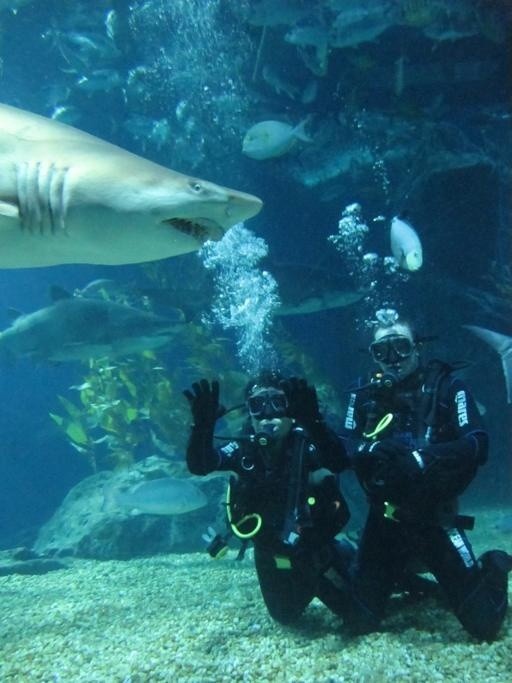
[183,378,226,429]
[374,437,407,466]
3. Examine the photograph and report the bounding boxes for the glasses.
[247,394,289,416]
[370,338,413,363]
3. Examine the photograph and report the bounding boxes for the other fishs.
[0,0,512,172]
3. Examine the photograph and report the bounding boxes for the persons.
[181,369,359,624]
[335,313,510,643]
